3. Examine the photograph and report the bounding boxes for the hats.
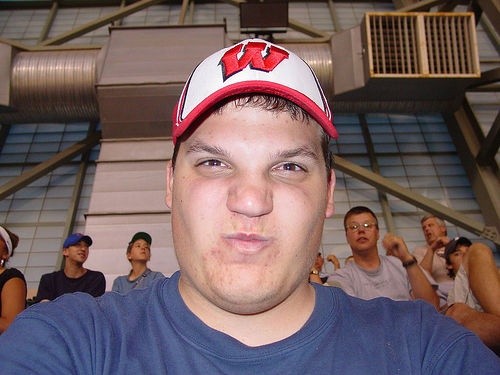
[445,237,472,256]
[1,226,13,257]
[62,233,93,249]
[128,232,152,245]
[172,38,338,145]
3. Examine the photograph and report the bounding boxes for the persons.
[0,224,27,334]
[0,39,500,375]
[322,206,440,310]
[35,234,106,302]
[112,232,165,294]
[413,214,455,283]
[437,236,500,347]
[309,252,340,285]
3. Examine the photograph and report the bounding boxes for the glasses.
[345,221,378,234]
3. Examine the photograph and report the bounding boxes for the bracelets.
[403,256,417,268]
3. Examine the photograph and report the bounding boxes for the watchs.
[311,270,319,275]
